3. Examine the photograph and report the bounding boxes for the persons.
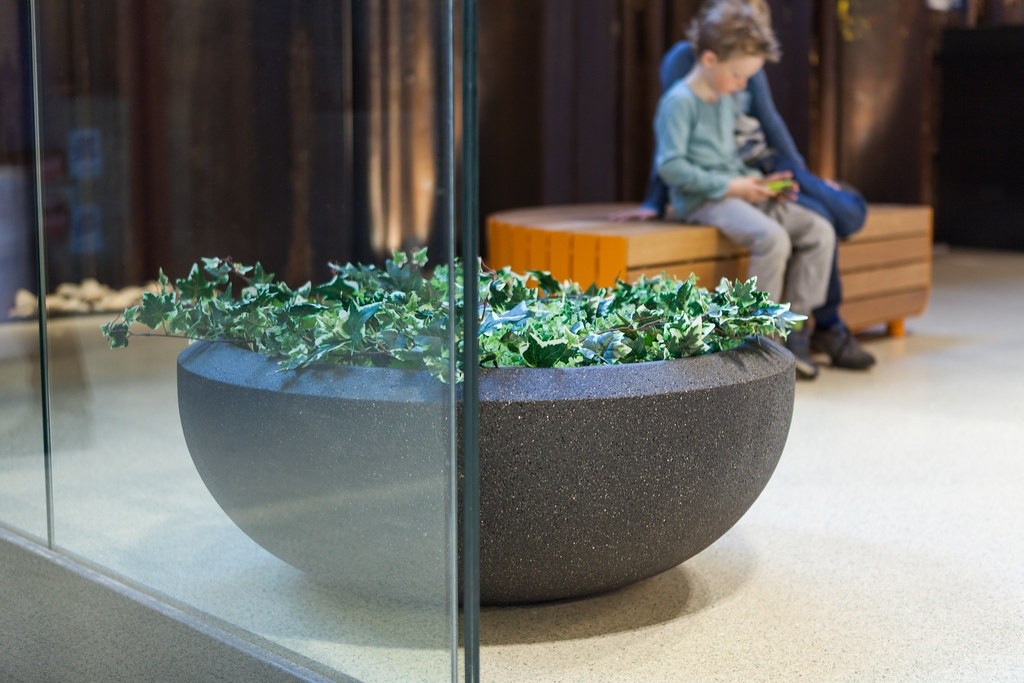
[607,0,878,382]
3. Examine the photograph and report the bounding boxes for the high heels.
[809,321,876,371]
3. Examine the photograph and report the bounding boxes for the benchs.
[487,201,933,346]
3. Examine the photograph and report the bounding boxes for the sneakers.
[760,320,818,379]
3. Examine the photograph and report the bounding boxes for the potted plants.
[100,247,810,609]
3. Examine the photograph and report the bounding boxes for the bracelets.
[741,181,747,198]
